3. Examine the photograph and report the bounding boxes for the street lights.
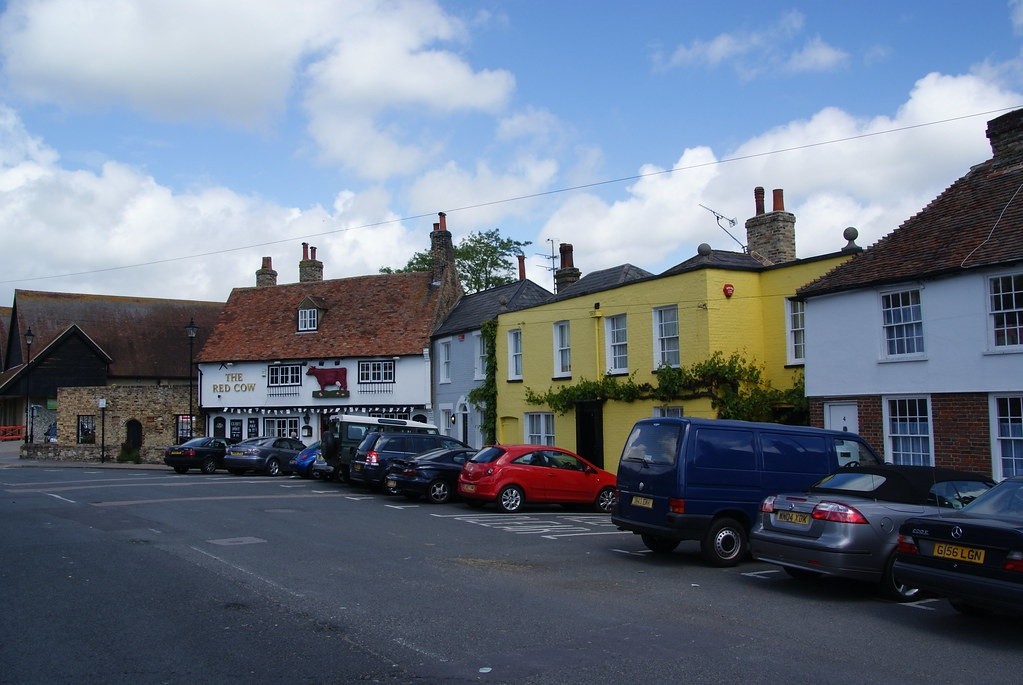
[24,323,35,445]
[185,316,200,440]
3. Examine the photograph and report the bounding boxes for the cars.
[288,440,322,478]
[223,435,308,476]
[891,473,1023,616]
[746,461,1023,602]
[382,445,493,505]
[312,450,346,483]
[456,443,618,513]
[163,436,240,474]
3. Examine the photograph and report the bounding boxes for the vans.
[610,416,890,570]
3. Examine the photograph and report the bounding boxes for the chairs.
[529,454,547,467]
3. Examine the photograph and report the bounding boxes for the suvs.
[348,430,474,498]
[319,413,442,484]
